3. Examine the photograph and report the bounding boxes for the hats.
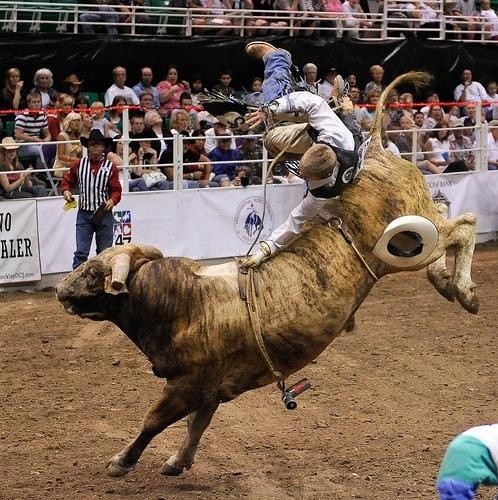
[0,136,22,150]
[487,120,498,132]
[372,215,439,268]
[62,74,84,86]
[165,130,196,144]
[80,128,113,149]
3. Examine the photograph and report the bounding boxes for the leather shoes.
[329,75,345,97]
[245,40,291,60]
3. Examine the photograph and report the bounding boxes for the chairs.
[0,1,186,34]
[0,88,153,194]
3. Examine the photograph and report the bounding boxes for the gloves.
[238,250,266,268]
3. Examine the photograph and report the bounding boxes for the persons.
[240,39,362,272]
[436,424,497,500]
[60,128,123,271]
[1,2,497,204]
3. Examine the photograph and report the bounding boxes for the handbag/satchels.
[142,159,168,188]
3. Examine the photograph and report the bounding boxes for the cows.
[54,69,481,479]
